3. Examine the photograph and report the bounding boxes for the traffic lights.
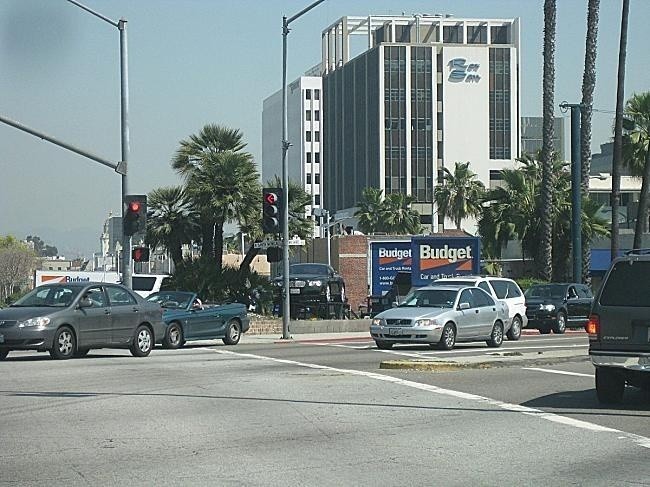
[124,201,140,234]
[263,187,283,232]
[132,247,149,261]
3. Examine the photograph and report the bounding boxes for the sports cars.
[145,291,250,349]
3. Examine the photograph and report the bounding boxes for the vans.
[587,248,650,395]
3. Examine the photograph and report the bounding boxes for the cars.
[273,263,345,302]
[370,275,594,349]
[0,282,168,360]
[133,274,173,298]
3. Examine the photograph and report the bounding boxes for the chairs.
[254,292,394,319]
[52,291,71,302]
[187,297,204,310]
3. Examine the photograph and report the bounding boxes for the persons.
[192,298,204,311]
